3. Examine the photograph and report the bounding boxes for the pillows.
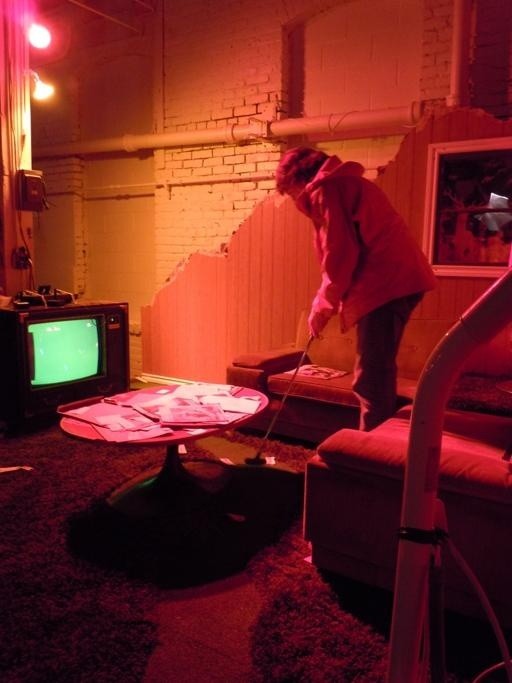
[447,374,511,416]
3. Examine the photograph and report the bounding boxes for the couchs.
[300,370,512,631]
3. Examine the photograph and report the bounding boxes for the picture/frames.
[417,136,512,281]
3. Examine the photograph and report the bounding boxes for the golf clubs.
[244,335,317,464]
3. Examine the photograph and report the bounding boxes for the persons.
[275,144,439,434]
[433,155,501,266]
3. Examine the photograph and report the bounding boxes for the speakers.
[17,169,44,211]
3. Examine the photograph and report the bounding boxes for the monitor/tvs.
[0,302,129,426]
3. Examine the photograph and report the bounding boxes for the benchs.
[222,340,420,451]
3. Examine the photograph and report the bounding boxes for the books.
[282,361,350,380]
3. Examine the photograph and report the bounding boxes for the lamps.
[26,68,55,100]
